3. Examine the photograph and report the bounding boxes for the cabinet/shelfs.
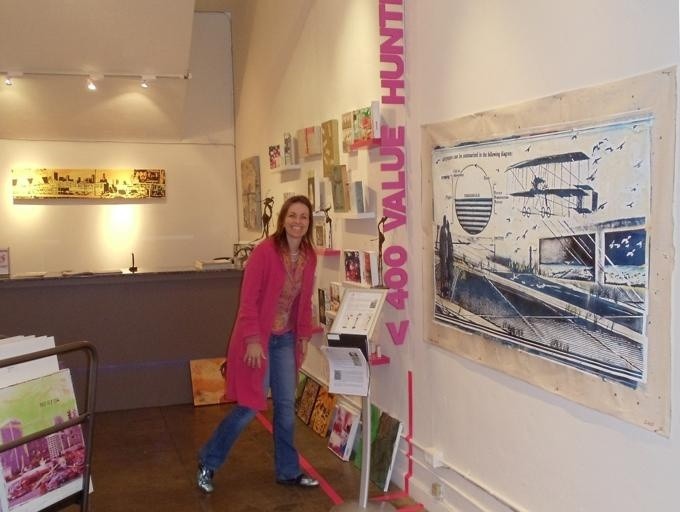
[270,137,382,320]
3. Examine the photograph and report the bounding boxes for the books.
[281,131,295,167]
[0,333,96,511]
[344,248,380,288]
[268,144,282,169]
[354,180,366,214]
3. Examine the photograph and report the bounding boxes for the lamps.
[0,71,192,91]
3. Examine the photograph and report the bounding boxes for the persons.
[438,216,448,291]
[444,219,454,287]
[193,195,320,495]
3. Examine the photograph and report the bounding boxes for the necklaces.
[289,249,299,257]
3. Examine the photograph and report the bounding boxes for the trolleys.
[1,340,98,512]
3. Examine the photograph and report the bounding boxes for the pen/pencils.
[132,253,134,267]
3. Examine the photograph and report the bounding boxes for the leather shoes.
[277,473,320,487]
[197,457,214,493]
